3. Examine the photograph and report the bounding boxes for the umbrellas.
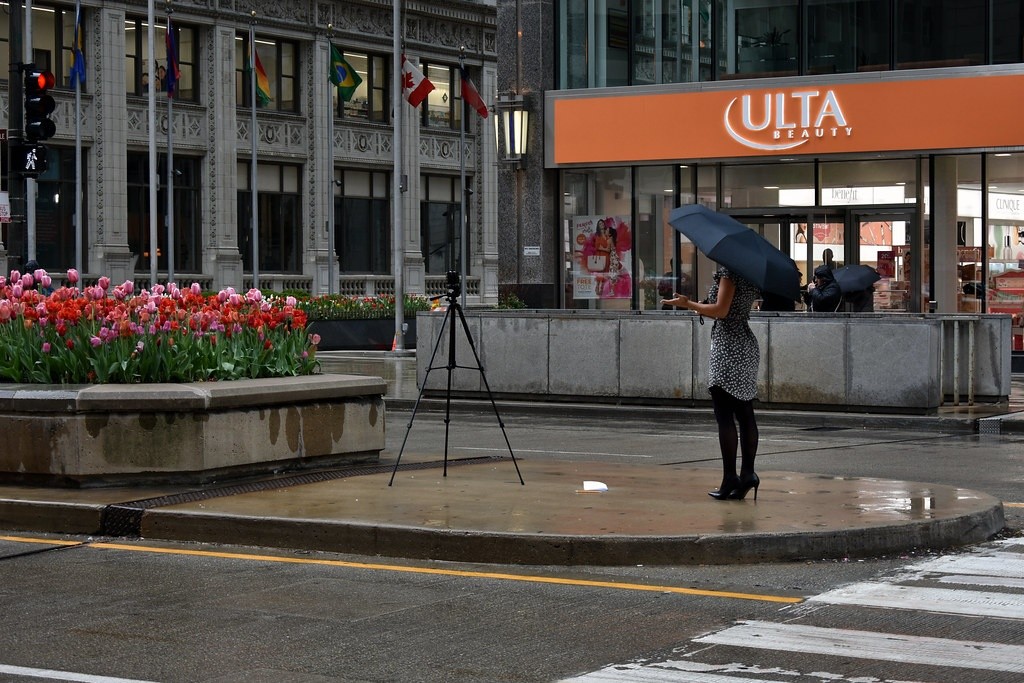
[666,202,803,309]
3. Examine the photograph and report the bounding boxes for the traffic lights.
[21,62,58,141]
[17,145,44,172]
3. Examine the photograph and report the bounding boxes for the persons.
[760,249,880,313]
[142,58,165,95]
[658,257,694,311]
[901,252,912,281]
[592,219,613,296]
[598,227,621,297]
[660,266,763,502]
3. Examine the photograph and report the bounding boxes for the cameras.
[446,271,461,297]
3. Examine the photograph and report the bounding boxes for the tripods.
[388,297,525,487]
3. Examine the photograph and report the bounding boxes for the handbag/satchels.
[587,249,607,272]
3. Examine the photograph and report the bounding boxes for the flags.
[460,62,489,119]
[70,0,89,94]
[248,27,271,108]
[165,16,181,98]
[400,51,435,108]
[329,41,362,102]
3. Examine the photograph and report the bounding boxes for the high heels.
[708,475,742,501]
[729,472,760,500]
